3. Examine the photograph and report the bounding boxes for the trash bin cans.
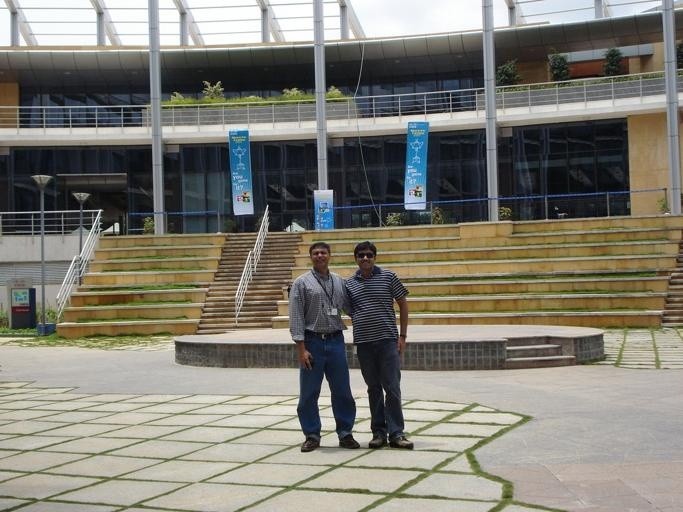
[11,288,36,330]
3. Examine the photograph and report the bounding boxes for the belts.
[314,330,343,338]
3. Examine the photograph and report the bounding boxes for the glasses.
[356,252,375,259]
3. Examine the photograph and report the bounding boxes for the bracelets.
[399,335,407,338]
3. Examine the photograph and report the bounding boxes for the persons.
[288,241,361,453]
[343,241,414,450]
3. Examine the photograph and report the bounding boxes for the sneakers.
[338,434,360,450]
[301,436,321,452]
[388,435,414,450]
[368,434,388,449]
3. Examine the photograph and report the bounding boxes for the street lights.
[30,174,52,336]
[68,191,92,287]
